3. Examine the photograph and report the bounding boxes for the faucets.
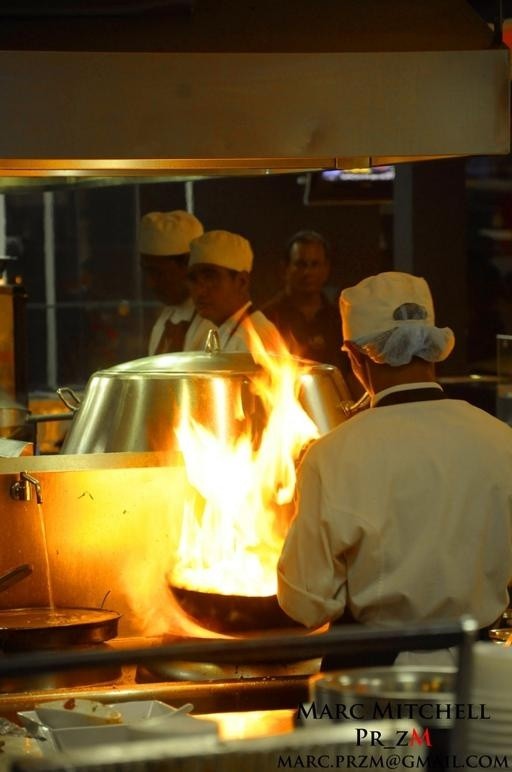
[18,471,43,504]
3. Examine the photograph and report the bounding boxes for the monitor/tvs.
[304,165,395,207]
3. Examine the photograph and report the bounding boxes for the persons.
[261,231,366,405]
[137,209,216,357]
[276,270,512,672]
[185,230,292,354]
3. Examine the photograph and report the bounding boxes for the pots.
[164,567,329,641]
[56,326,372,456]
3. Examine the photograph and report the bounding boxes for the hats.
[186,229,255,276]
[338,270,435,348]
[134,207,205,259]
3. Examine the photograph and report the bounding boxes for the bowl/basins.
[15,700,218,756]
